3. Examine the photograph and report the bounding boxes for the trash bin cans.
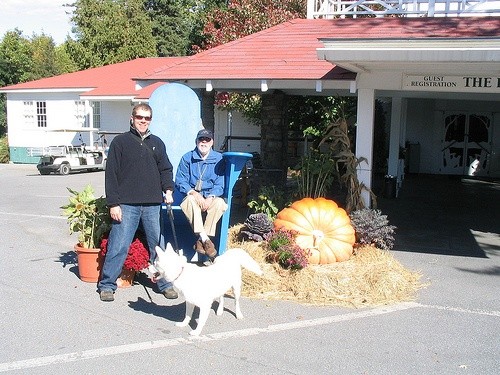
[384,175,396,199]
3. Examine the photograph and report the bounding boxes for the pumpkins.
[273,198,356,265]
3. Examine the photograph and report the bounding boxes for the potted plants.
[59,183,112,283]
[99,238,149,271]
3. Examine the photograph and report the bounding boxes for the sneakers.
[202,239,217,258]
[193,240,206,255]
[162,288,178,299]
[100,290,114,301]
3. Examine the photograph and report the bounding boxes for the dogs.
[147,242,263,336]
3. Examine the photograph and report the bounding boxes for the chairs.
[240,151,283,206]
[49,144,80,155]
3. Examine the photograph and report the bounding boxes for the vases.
[115,269,136,288]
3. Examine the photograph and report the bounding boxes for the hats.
[197,129,213,139]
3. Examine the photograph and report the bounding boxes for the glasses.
[132,114,151,121]
[198,137,211,142]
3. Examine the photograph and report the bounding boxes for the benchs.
[104,83,253,257]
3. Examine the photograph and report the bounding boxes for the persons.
[175,129,227,258]
[98,103,178,301]
